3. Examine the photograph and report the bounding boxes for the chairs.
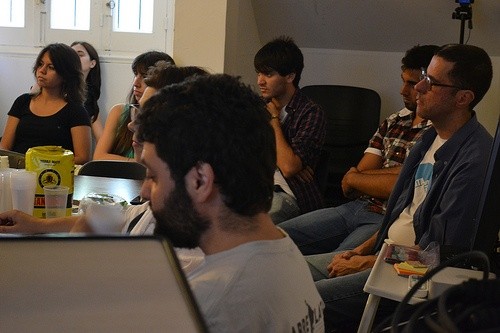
[78,160,146,181]
[300,82,382,168]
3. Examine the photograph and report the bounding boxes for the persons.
[250,37,496,333]
[0,41,221,274]
[135,74,326,333]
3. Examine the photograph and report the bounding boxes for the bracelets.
[271,116,279,119]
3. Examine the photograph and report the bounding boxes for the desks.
[73,175,144,207]
[355,239,429,333]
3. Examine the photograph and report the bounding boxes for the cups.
[0,168,37,216]
[43,185,70,220]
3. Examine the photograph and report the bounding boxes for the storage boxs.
[429,266,496,298]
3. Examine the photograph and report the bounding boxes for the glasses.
[419,67,477,97]
[129,104,140,121]
